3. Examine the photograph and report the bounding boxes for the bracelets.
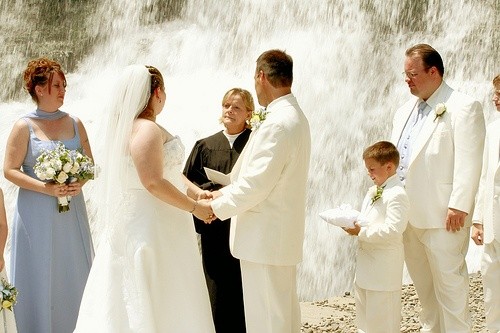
[189,203,197,215]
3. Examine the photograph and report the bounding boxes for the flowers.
[0,275,20,313]
[246,107,272,133]
[369,183,387,206]
[32,140,101,213]
[431,103,448,125]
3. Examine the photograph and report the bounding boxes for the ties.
[412,102,428,128]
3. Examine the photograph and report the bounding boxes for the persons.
[0,58,93,333]
[213,50,311,333]
[391,44,486,333]
[182,88,257,333]
[93,65,218,333]
[0,190,17,333]
[470,76,500,332]
[338,141,410,333]
